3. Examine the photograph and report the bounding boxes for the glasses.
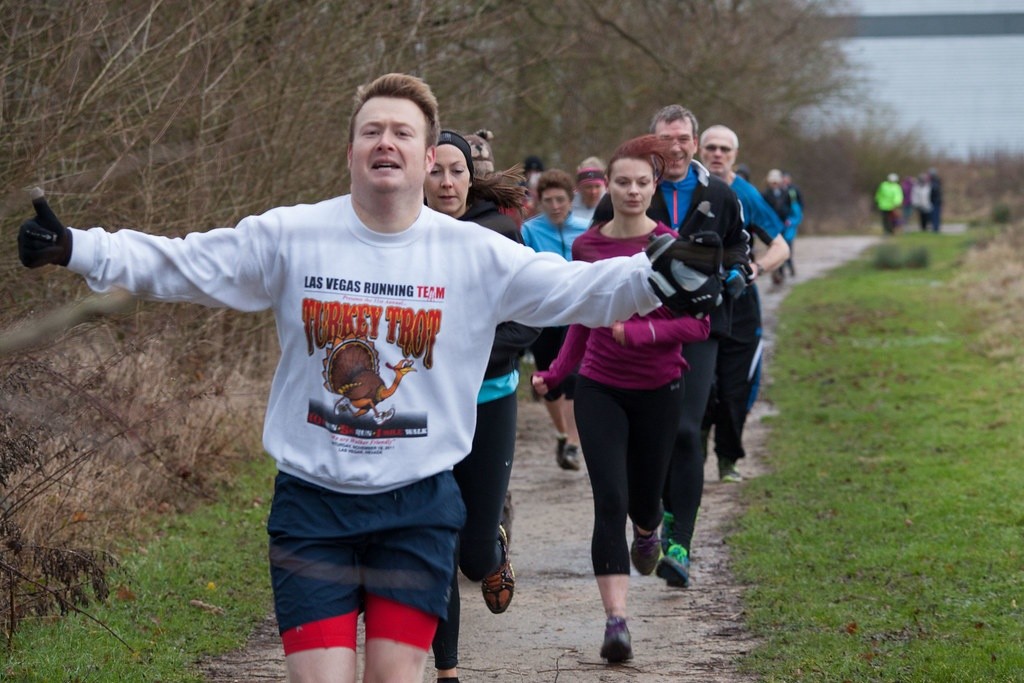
[704,145,732,153]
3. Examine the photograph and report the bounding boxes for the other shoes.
[557,434,581,472]
[716,458,743,482]
[503,491,512,547]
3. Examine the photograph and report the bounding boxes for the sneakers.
[656,509,691,588]
[630,521,660,576]
[600,616,635,664]
[481,522,517,615]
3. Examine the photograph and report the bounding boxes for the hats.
[522,155,545,173]
[463,128,497,178]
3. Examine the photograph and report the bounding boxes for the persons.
[15,73,728,683]
[424,104,942,683]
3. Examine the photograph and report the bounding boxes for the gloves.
[17,186,73,269]
[646,199,722,321]
[719,261,749,302]
